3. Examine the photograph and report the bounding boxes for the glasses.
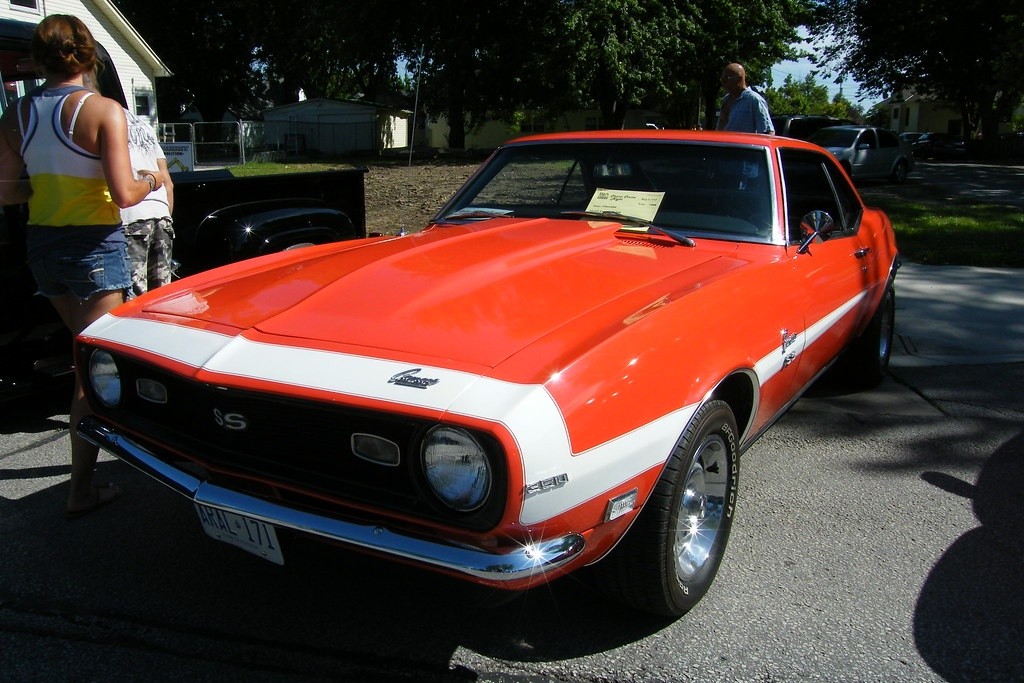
[719,76,739,83]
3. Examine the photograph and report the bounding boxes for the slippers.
[65,482,123,520]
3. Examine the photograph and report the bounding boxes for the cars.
[899,132,924,144]
[910,132,969,161]
[72,130,904,629]
[805,125,916,185]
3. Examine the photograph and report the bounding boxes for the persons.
[657,123,702,131]
[88,84,175,305]
[713,62,776,193]
[975,131,984,141]
[0,14,169,518]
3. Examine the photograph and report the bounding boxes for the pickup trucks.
[0,17,368,418]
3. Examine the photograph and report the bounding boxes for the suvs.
[771,112,861,143]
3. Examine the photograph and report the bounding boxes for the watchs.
[149,182,153,192]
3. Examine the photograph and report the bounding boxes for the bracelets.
[143,176,155,186]
[144,172,157,191]
